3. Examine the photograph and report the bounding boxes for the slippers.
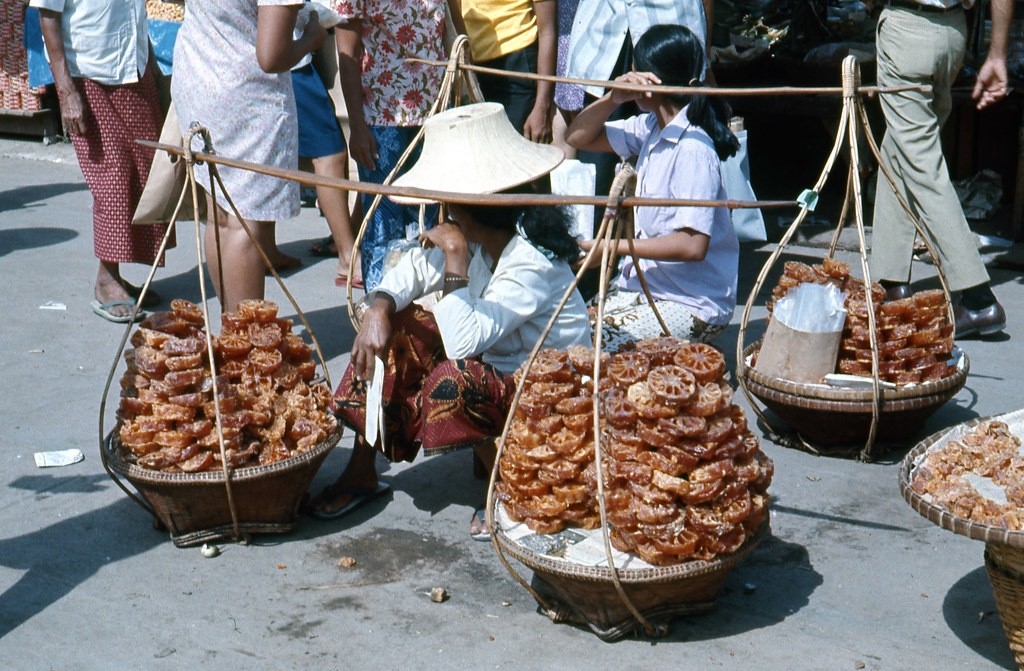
[470,505,491,540]
[91,297,145,322]
[310,480,390,520]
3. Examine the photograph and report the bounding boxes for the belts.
[885,0,961,13]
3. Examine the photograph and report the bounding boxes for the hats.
[388,102,565,204]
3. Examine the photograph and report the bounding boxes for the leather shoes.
[953,302,1007,338]
[881,285,912,305]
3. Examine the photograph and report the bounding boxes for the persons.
[22,1,1012,540]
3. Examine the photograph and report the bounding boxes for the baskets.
[103,426,342,547]
[984,542,1024,671]
[492,492,772,638]
[899,411,1024,548]
[742,337,966,462]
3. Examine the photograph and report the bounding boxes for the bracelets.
[444,277,469,281]
